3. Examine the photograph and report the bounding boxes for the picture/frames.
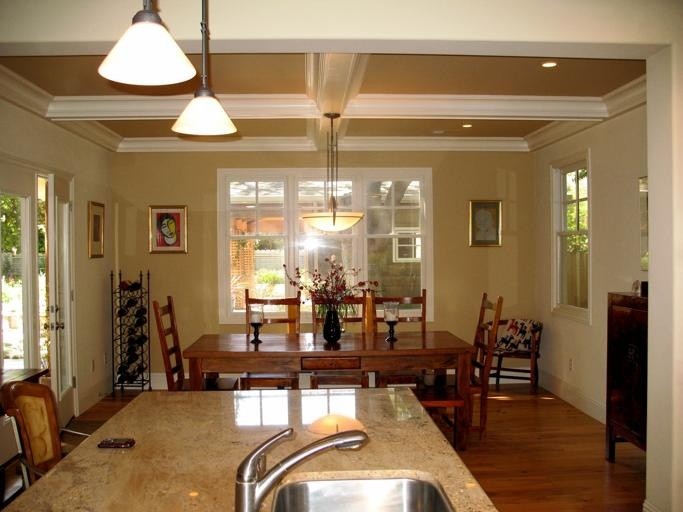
[84,199,105,258]
[146,204,189,255]
[467,198,502,249]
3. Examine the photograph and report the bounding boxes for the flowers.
[280,256,380,323]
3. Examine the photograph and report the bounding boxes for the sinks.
[273,477,451,512]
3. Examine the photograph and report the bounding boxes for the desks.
[1,368,48,410]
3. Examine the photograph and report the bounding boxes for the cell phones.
[98,438,135,448]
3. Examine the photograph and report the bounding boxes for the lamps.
[299,111,365,232]
[95,1,240,141]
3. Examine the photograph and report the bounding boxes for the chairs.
[0,379,92,511]
[477,316,545,392]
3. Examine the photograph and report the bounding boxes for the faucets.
[234,428,369,512]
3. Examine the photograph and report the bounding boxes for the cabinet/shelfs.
[603,292,647,464]
